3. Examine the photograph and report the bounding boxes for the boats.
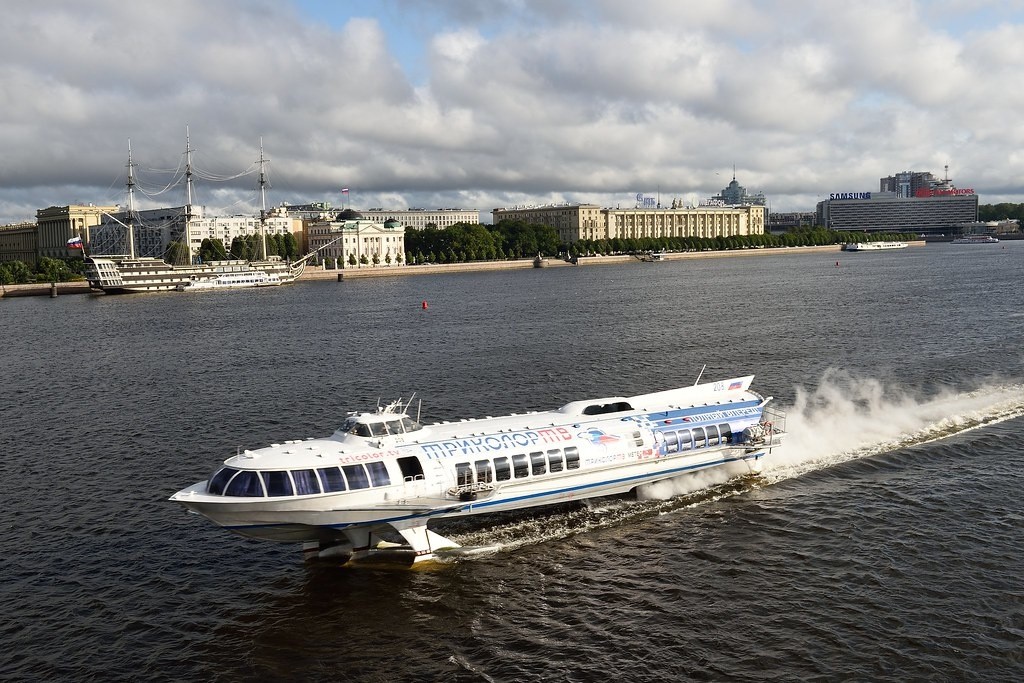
[534,251,549,268]
[951,235,1000,243]
[653,253,665,262]
[67,120,344,293]
[846,241,908,251]
[169,362,790,566]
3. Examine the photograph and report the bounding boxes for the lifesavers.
[763,421,774,436]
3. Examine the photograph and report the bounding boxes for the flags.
[67,236,82,248]
[341,188,349,194]
